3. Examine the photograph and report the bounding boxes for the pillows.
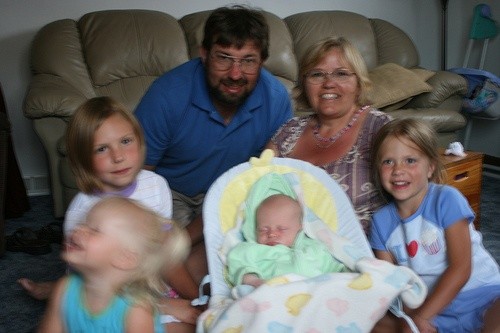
[367,61,436,107]
[384,68,437,113]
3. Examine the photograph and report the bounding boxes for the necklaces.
[312,105,371,150]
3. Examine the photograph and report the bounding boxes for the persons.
[368,115,500,332]
[266,35,399,240]
[17,96,207,333]
[129,5,295,299]
[225,194,353,290]
[40,196,194,333]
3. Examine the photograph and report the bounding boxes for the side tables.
[439,144,485,231]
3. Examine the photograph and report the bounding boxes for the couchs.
[22,5,470,219]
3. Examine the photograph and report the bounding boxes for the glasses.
[207,49,263,76]
[303,67,357,85]
